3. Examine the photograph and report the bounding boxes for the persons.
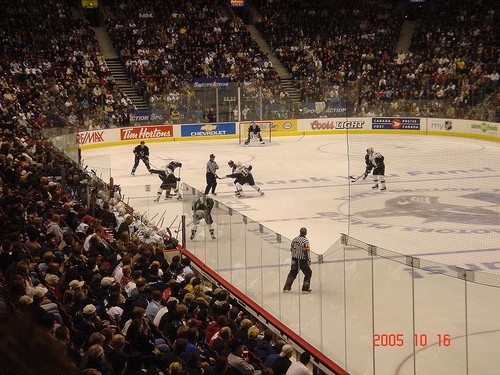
[244,120,264,146]
[100,0,294,124]
[0,1,138,140]
[361,149,378,181]
[246,0,500,123]
[368,147,388,191]
[1,139,315,375]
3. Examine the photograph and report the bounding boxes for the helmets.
[228,161,233,164]
[367,148,374,155]
[140,141,145,144]
[236,161,242,166]
[170,162,175,168]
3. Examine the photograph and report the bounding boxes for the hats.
[210,154,216,158]
[240,319,252,327]
[69,280,84,289]
[101,276,114,287]
[279,344,293,358]
[33,288,48,299]
[83,304,96,314]
[89,333,106,344]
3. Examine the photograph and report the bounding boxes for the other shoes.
[213,193,217,195]
[212,236,216,239]
[283,290,290,293]
[235,191,239,193]
[131,172,135,175]
[177,197,183,200]
[166,196,172,198]
[261,192,264,195]
[302,289,312,294]
[154,199,160,204]
[238,196,245,198]
[190,235,194,239]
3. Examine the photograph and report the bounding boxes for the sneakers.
[372,186,379,190]
[381,188,387,191]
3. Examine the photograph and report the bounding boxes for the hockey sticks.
[149,213,158,222]
[174,168,180,193]
[175,220,181,240]
[215,174,225,179]
[161,214,179,236]
[155,210,166,227]
[350,172,365,182]
[142,211,148,217]
[174,221,193,233]
[77,161,103,183]
[141,157,158,170]
